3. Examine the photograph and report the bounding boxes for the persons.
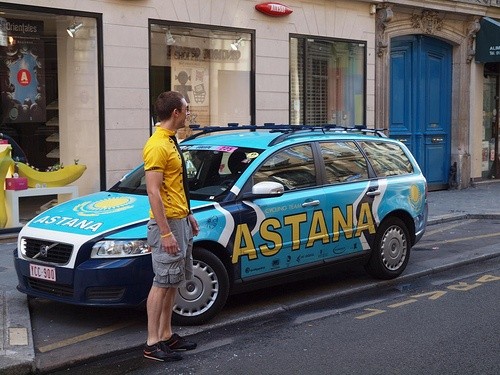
[143,91,201,363]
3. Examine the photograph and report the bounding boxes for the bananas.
[14,161,87,188]
[0,146,12,230]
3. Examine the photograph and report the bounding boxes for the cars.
[12,123,429,328]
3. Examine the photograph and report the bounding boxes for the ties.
[171,136,190,210]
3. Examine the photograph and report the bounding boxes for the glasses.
[185,105,189,112]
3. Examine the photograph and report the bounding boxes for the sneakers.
[165,333,197,352]
[144,340,183,362]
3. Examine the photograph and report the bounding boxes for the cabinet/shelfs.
[46,101,59,158]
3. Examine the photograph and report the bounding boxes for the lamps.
[231,36,244,51]
[164,27,174,45]
[67,16,82,38]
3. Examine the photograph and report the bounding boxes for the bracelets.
[160,231,172,239]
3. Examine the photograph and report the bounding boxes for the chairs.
[212,150,251,192]
